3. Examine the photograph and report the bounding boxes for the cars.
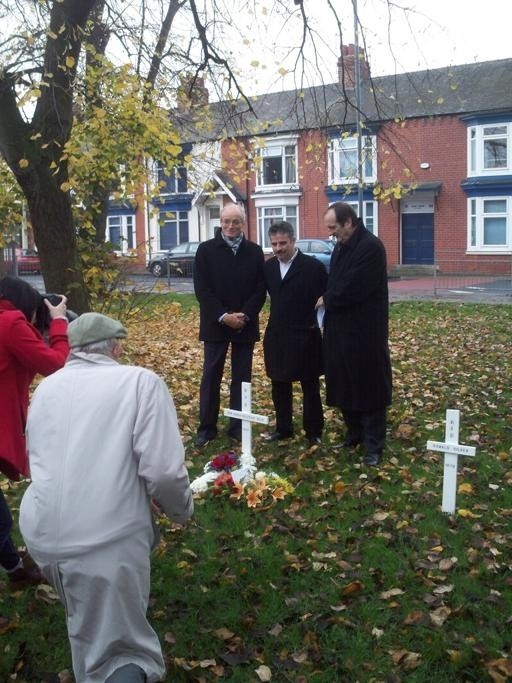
[148,241,203,278]
[2,245,41,275]
[298,237,338,274]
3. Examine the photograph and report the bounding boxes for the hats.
[65,311,129,348]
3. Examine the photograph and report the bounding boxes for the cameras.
[41,292,78,326]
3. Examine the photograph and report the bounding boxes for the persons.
[20,310,194,680]
[265,220,329,449]
[1,272,69,581]
[315,201,392,467]
[194,201,266,448]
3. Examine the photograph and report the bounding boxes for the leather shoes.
[337,443,361,459]
[7,559,26,583]
[264,431,296,443]
[363,448,384,466]
[304,433,321,449]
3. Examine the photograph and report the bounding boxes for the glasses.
[221,218,245,230]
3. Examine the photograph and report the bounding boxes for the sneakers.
[194,434,214,449]
[230,436,242,445]
